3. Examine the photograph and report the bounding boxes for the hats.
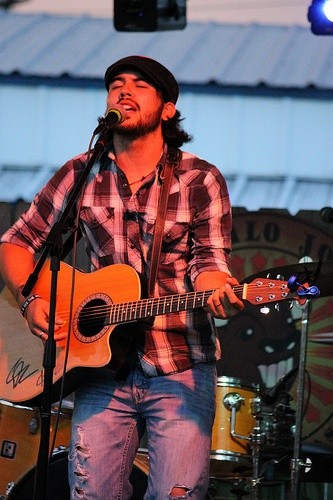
[104,55,179,105]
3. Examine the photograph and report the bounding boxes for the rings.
[38,331,44,339]
[215,303,221,308]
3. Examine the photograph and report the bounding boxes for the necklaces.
[128,170,155,186]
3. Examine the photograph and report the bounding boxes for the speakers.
[114,0,187,32]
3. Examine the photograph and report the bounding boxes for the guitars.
[1,259,319,402]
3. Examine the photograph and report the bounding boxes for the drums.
[1,400,71,497]
[211,375,261,475]
[5,448,188,499]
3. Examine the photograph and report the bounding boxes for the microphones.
[94,104,126,134]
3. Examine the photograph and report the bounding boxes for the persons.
[0,56,246,499]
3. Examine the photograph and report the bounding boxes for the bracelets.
[19,293,42,317]
